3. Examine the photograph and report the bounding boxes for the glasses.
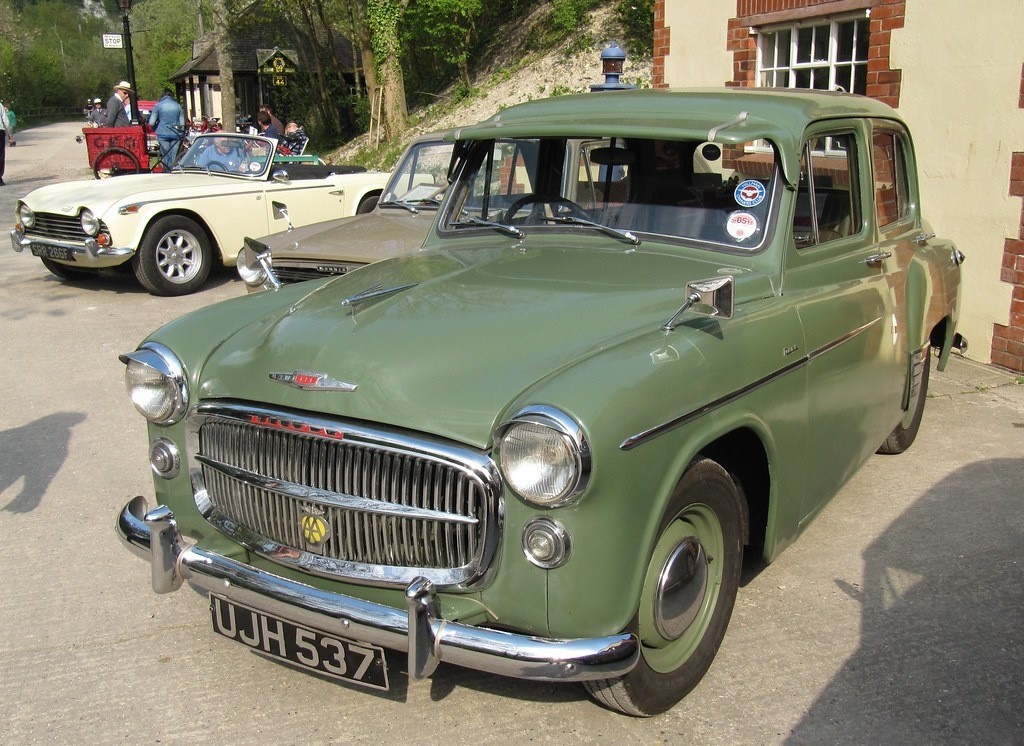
[120,89,128,94]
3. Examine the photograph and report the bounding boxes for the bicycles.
[84,109,92,121]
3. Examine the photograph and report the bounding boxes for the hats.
[114,81,135,93]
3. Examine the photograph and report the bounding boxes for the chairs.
[793,186,850,250]
[615,159,703,240]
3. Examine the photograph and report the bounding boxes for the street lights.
[116,0,141,126]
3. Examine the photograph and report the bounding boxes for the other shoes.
[0,177,6,186]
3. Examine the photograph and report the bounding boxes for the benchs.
[249,162,366,180]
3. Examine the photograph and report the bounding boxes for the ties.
[99,109,100,114]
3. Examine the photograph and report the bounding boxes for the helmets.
[87,99,91,101]
[94,98,102,103]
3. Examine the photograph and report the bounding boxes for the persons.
[4,105,16,147]
[260,104,284,133]
[257,111,283,146]
[84,99,94,116]
[124,97,131,122]
[281,123,307,163]
[105,81,135,127]
[198,130,250,172]
[0,103,9,186]
[149,88,185,173]
[90,98,107,123]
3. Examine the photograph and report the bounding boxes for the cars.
[115,47,967,721]
[236,123,632,298]
[137,100,160,151]
[10,132,434,297]
